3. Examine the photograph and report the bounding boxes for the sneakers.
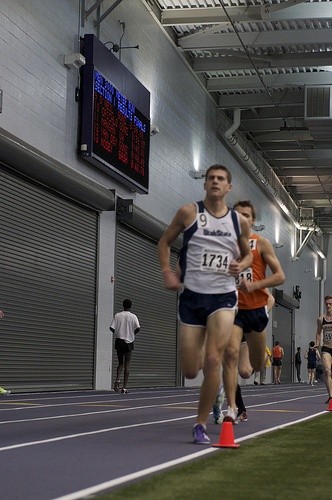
[213,397,248,425]
[191,422,211,445]
[325,397,332,411]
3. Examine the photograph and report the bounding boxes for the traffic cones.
[326,398,332,411]
[212,420,241,448]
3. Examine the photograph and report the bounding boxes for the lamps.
[64,52,86,70]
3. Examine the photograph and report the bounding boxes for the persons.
[158,165,253,444]
[109,299,140,395]
[212,200,332,424]
